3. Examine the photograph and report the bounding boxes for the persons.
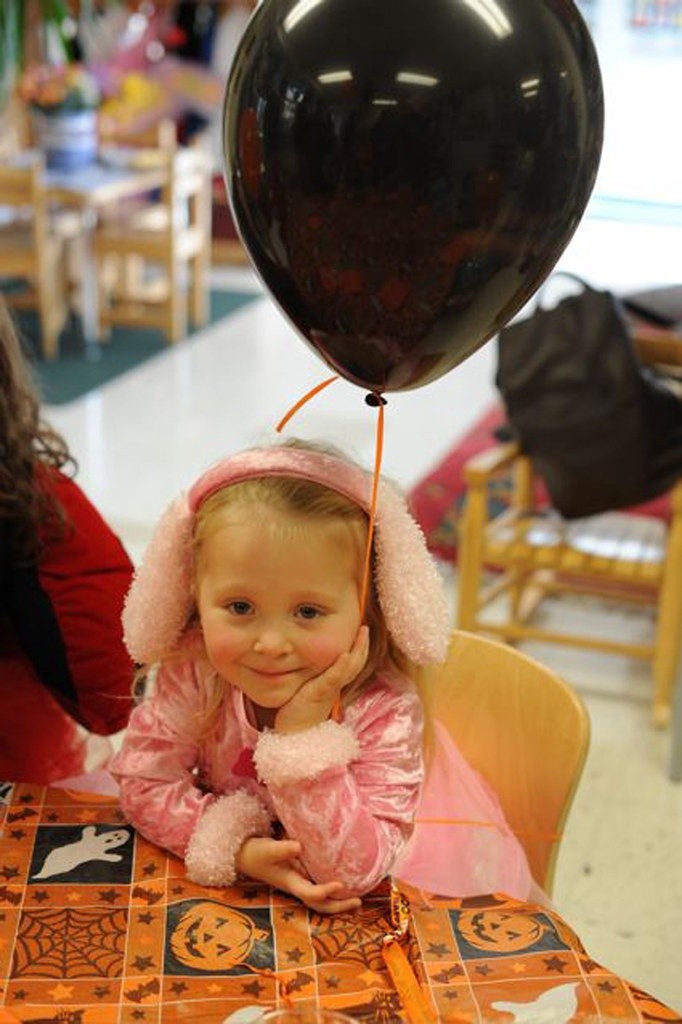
[49,439,557,914]
[0,298,147,789]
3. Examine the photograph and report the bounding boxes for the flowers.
[18,58,103,119]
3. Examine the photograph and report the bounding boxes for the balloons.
[221,0,604,407]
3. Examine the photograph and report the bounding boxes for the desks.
[47,144,173,342]
[1,778,682,1024]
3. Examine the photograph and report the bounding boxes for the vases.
[31,111,99,177]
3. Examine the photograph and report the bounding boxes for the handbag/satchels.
[494,270,682,520]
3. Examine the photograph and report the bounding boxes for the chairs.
[422,615,591,895]
[0,119,212,363]
[457,327,681,731]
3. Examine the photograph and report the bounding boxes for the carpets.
[2,273,262,409]
